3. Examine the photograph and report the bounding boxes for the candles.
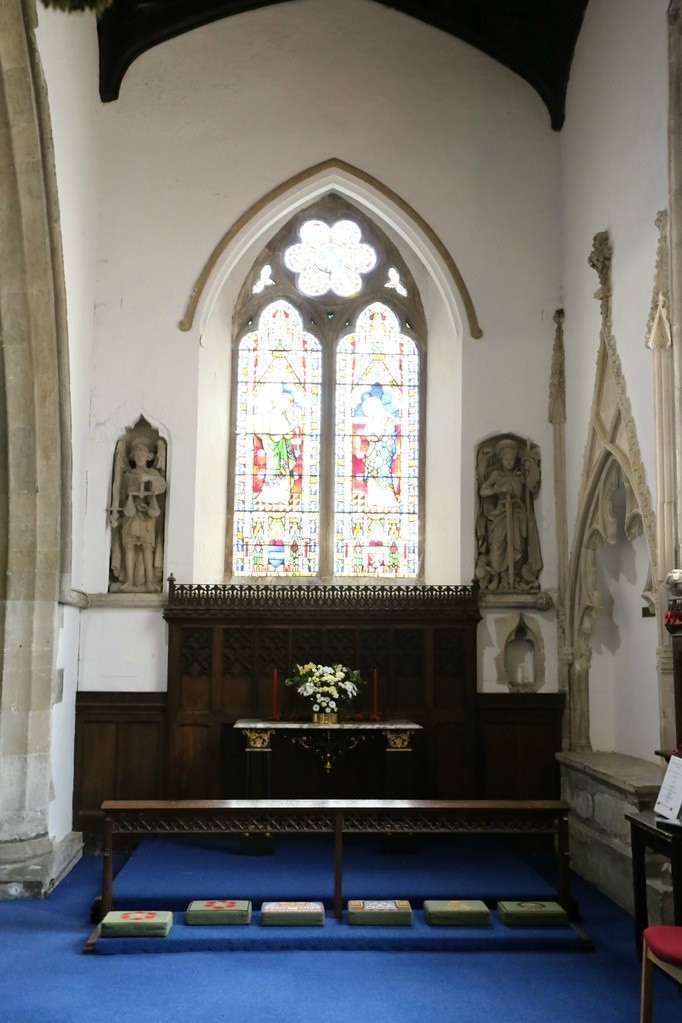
[373,672,378,721]
[274,671,278,721]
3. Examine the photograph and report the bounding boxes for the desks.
[101,800,571,931]
[624,810,682,970]
[233,717,422,796]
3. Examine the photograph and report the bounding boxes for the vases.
[313,712,338,724]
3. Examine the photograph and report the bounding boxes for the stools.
[640,925,682,1023]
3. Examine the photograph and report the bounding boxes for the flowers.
[285,663,366,711]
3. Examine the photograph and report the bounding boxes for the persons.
[479,439,539,589]
[118,437,166,590]
